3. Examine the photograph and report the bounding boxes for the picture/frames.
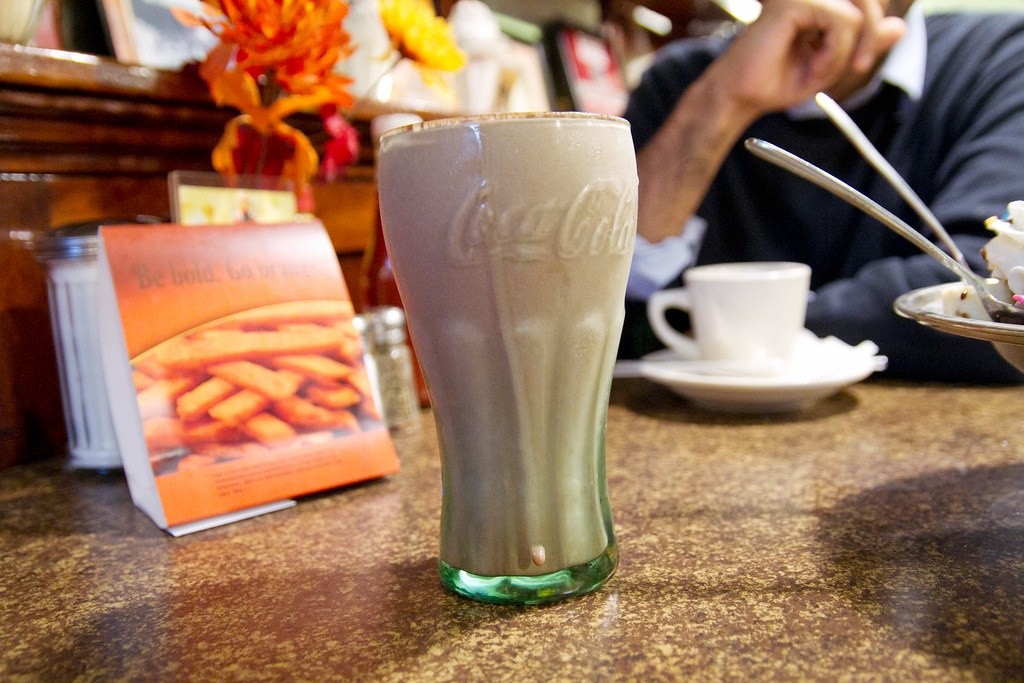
[537,17,633,118]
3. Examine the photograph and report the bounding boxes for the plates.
[642,347,872,414]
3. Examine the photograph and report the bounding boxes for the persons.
[605,0,1024,391]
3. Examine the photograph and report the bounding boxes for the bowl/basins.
[892,278,1024,376]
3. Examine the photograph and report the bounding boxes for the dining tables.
[0,358,1024,683]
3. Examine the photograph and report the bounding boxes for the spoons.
[745,138,1024,326]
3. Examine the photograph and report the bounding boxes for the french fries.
[129,312,379,476]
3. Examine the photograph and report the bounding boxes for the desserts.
[942,199,1024,324]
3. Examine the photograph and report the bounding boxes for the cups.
[646,260,812,361]
[376,111,639,608]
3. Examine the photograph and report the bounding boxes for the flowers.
[168,0,467,215]
